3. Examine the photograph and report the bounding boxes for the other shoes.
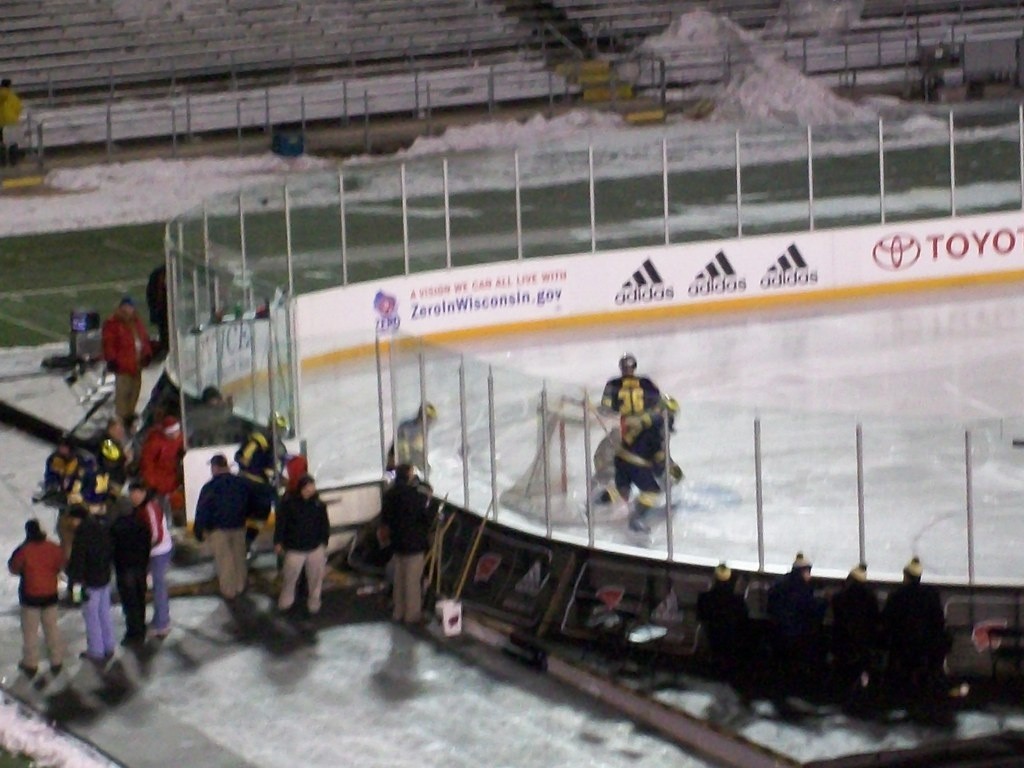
[120,629,144,645]
[80,651,113,664]
[18,659,38,678]
[51,663,62,674]
[624,518,653,540]
[146,622,168,636]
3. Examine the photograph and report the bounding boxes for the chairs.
[37,310,103,394]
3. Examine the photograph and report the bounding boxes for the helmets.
[418,401,438,420]
[656,396,679,416]
[618,353,636,371]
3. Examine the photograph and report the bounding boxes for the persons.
[8,521,64,675]
[382,464,435,627]
[66,503,114,661]
[592,353,684,534]
[102,297,151,421]
[386,403,436,473]
[192,455,249,601]
[235,413,288,554]
[111,496,151,646]
[44,386,233,519]
[0,79,24,167]
[274,474,332,620]
[696,554,953,684]
[129,482,172,635]
[146,262,169,352]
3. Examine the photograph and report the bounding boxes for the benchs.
[0,0,1024,150]
